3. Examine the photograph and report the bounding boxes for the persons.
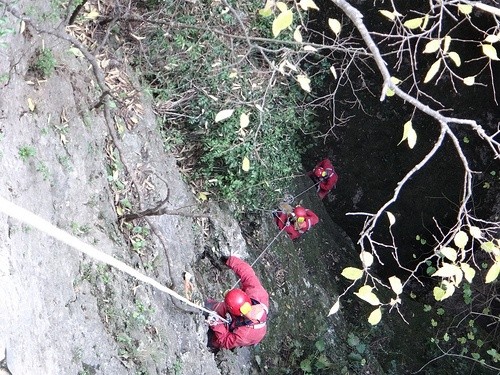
[274,203,320,240]
[307,158,338,201]
[204,255,270,351]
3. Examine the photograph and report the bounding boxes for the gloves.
[220,255,229,264]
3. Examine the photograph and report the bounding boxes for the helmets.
[293,206,307,221]
[225,288,251,316]
[314,167,325,178]
